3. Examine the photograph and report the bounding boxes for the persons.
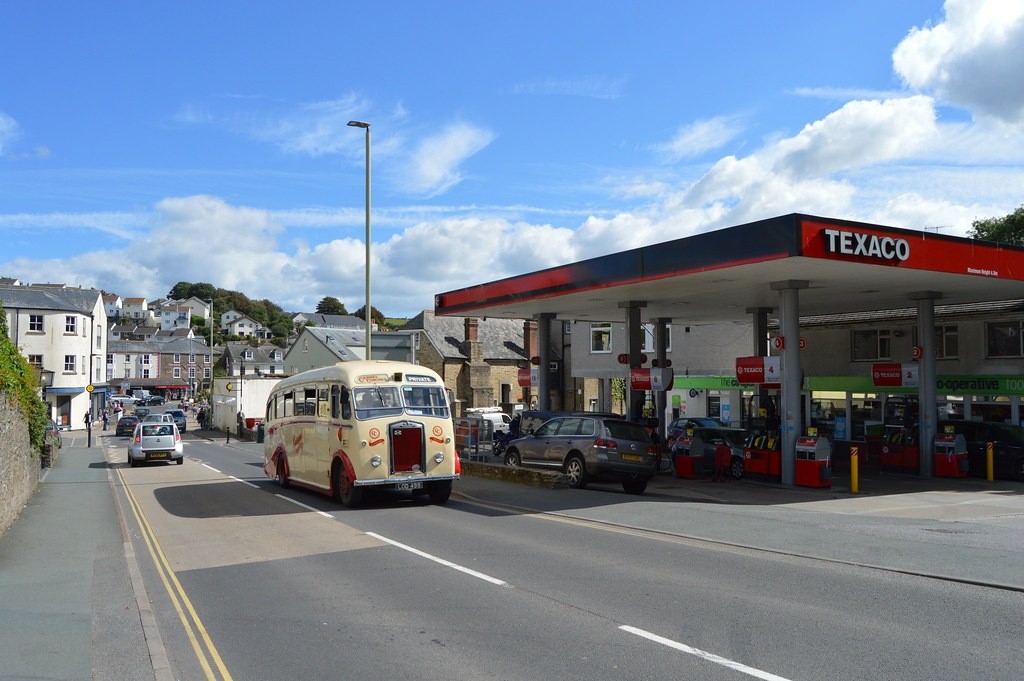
[355,392,383,419]
[85,402,122,431]
[185,398,206,431]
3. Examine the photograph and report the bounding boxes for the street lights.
[236,354,244,439]
[345,118,373,362]
[124,338,129,379]
[89,353,97,415]
[203,297,215,432]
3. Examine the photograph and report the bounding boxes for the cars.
[670,426,755,480]
[667,416,728,453]
[135,394,165,407]
[114,415,141,438]
[107,394,141,405]
[45,419,64,450]
[130,407,187,434]
[126,421,184,467]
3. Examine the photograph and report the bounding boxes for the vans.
[261,361,460,510]
[464,407,513,441]
[132,389,154,399]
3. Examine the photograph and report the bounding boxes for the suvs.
[504,410,661,495]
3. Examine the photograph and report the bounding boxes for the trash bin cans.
[934,433,969,478]
[794,436,833,488]
[675,436,704,479]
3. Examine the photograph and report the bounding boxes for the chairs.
[144,430,151,436]
[159,427,169,435]
